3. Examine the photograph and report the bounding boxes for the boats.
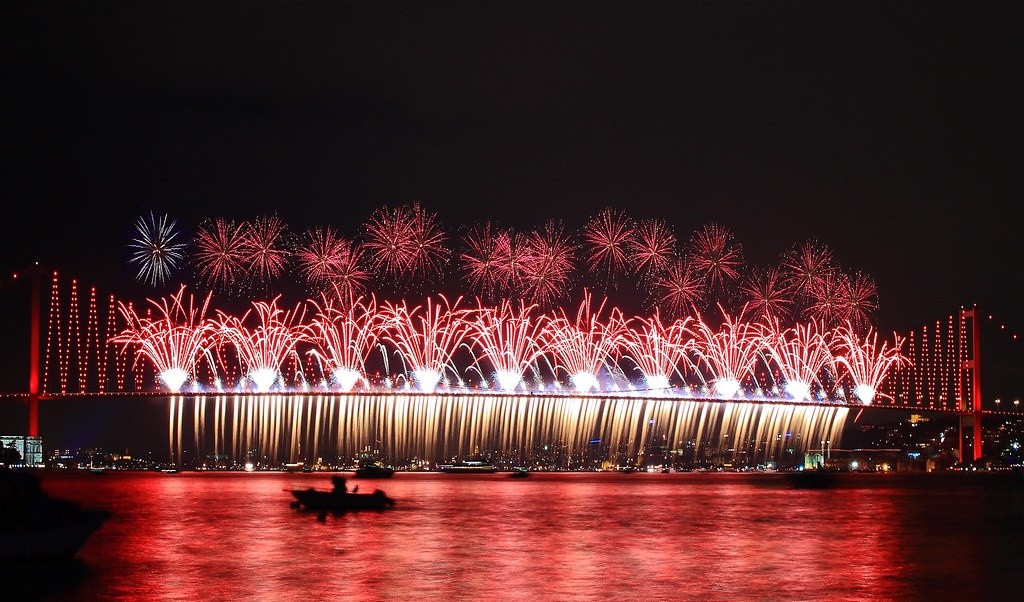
[623,467,633,473]
[356,466,394,476]
[662,469,670,473]
[284,488,395,512]
[436,461,499,472]
